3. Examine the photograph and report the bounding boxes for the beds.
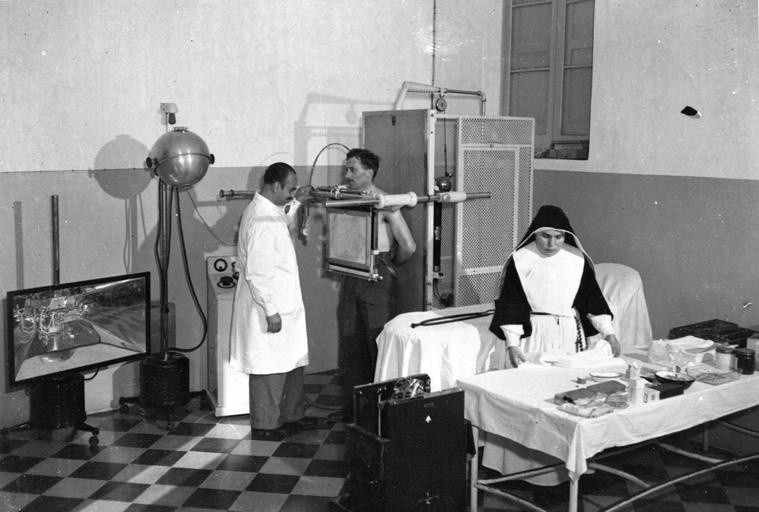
[378,263,642,388]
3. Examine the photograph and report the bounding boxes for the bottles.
[715,346,755,376]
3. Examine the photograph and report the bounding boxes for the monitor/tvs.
[8,271,151,384]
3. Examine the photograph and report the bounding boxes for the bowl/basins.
[655,371,696,390]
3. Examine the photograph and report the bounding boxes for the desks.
[460,333,759,512]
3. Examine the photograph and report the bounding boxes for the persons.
[488,203,624,509]
[230,160,331,441]
[323,148,419,427]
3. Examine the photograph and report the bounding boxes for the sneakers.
[251,415,319,441]
[328,404,379,422]
